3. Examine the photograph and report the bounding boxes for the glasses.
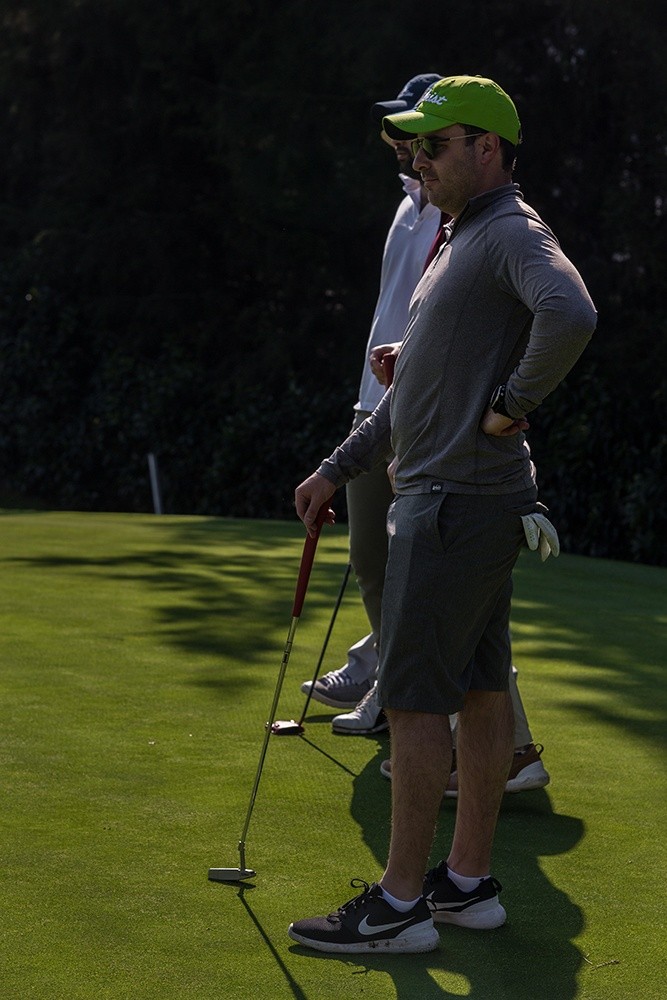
[410,133,481,160]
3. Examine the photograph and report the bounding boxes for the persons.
[299,128,442,734]
[288,76,598,957]
[370,74,551,801]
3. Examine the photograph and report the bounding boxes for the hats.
[381,129,396,148]
[382,75,522,146]
[372,74,445,122]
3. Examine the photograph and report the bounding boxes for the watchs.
[491,385,515,420]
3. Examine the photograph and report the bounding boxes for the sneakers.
[301,664,371,709]
[424,860,507,929]
[332,686,389,734]
[380,758,392,778]
[288,879,441,953]
[442,744,550,797]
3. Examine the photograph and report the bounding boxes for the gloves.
[522,512,560,562]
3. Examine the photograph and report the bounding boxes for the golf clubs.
[208,507,332,883]
[264,559,352,736]
